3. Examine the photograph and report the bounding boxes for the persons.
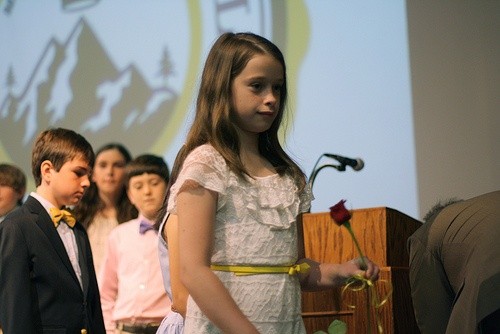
[71,143,135,282]
[153,144,188,334]
[167,31,380,334]
[0,164,27,223]
[0,128,108,334]
[98,154,170,334]
[406,190,500,334]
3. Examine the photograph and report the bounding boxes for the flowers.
[330,199,395,334]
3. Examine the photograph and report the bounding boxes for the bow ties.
[50,207,76,228]
[139,220,160,235]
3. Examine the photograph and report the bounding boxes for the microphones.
[323,153,365,172]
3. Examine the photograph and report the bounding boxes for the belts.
[211,263,312,276]
[116,323,159,334]
